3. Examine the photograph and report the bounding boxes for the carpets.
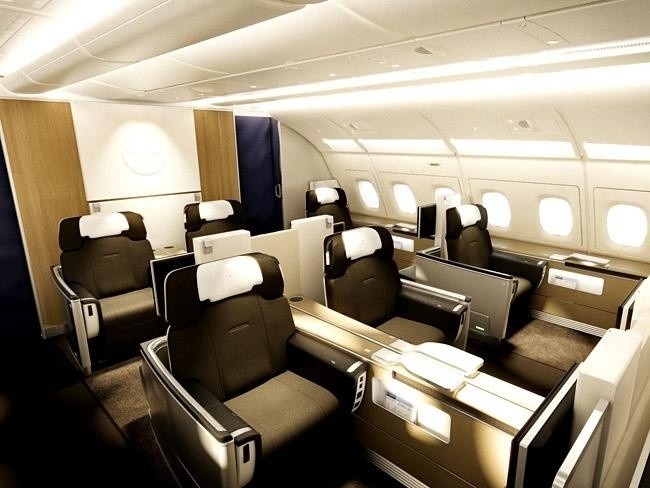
[505,316,602,372]
[84,358,155,429]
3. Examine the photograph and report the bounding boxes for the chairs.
[49,212,171,376]
[140,252,368,488]
[183,199,249,254]
[322,226,471,350]
[416,204,548,345]
[305,188,353,233]
